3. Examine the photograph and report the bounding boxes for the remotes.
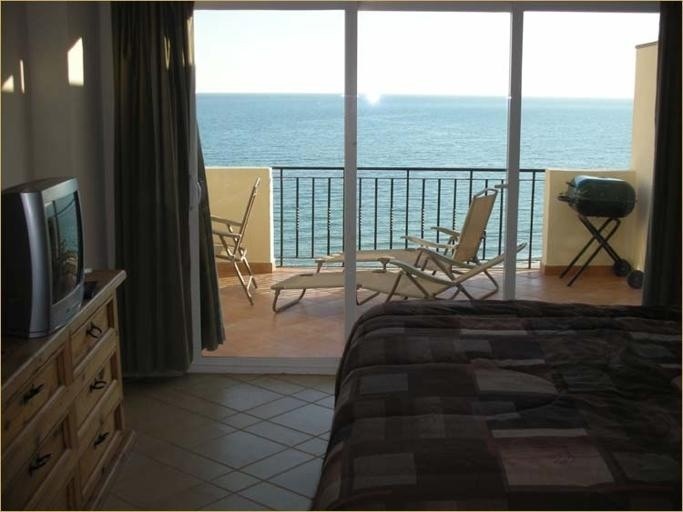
[84,280,97,297]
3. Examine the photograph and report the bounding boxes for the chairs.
[210,177,262,306]
[271,188,527,313]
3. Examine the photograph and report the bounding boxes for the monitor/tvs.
[1,177,86,339]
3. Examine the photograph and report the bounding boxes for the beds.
[313,301,681,510]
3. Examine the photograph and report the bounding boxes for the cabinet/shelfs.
[2,270,137,511]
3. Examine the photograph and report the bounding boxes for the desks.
[558,214,632,287]
